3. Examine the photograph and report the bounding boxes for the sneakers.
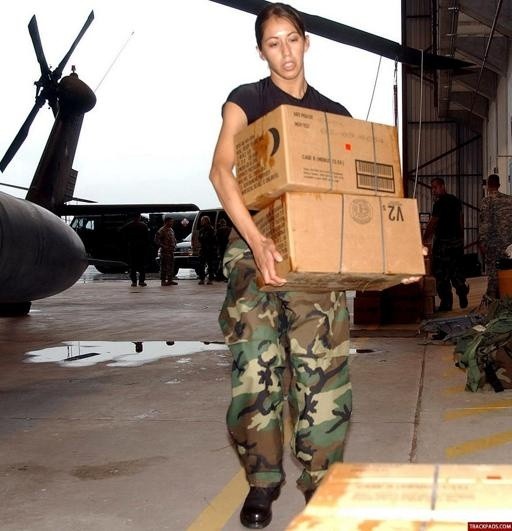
[130,280,147,288]
[198,279,214,286]
[161,279,178,287]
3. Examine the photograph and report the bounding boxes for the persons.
[209,2,429,530]
[155,216,178,286]
[119,211,151,288]
[421,177,469,313]
[197,216,219,285]
[215,219,231,256]
[477,174,511,307]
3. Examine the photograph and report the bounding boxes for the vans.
[191,208,232,256]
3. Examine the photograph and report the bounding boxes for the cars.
[156,232,208,277]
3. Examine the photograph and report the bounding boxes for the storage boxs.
[233,102,430,295]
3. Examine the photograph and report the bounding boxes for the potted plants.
[495,255,512,300]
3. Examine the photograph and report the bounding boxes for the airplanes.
[0,10,96,317]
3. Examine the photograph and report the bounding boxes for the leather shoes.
[434,296,469,313]
[239,481,281,530]
[304,486,317,505]
